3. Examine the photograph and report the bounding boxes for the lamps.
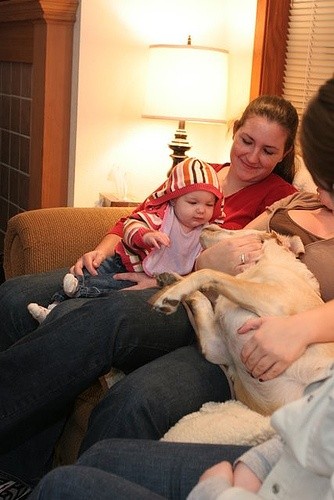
[140,34,230,178]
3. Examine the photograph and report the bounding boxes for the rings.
[240,254,246,264]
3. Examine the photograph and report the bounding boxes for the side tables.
[100,191,143,207]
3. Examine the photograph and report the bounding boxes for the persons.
[23,73,334,500]
[0,95,299,474]
[0,192,334,461]
[28,156,226,326]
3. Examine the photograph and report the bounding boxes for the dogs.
[148,224,333,446]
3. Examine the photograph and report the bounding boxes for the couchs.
[4,206,142,465]
